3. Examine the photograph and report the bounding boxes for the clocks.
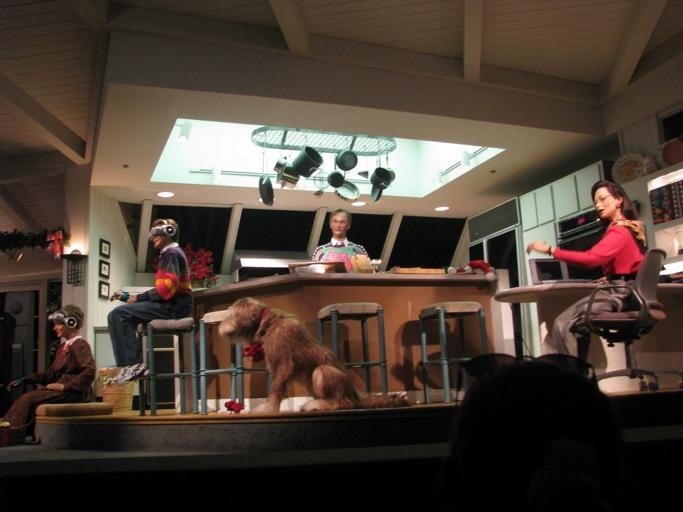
[613,152,645,185]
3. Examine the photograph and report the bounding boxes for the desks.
[493,280,597,354]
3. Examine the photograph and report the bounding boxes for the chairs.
[570,248,683,395]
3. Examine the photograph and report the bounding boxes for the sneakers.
[117,361,149,386]
[113,366,131,381]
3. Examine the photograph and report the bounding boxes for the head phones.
[162,221,176,237]
[63,314,78,329]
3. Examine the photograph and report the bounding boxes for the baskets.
[99,367,135,412]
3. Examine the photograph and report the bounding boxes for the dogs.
[217,296,410,413]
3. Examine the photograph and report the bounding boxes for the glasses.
[591,193,611,208]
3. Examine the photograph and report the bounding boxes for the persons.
[449,361,634,512]
[527,181,650,379]
[1,305,96,446]
[311,208,373,273]
[107,219,193,385]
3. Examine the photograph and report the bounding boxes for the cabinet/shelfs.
[516,157,612,359]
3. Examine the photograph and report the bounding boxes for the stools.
[137,316,195,415]
[418,301,489,404]
[200,310,274,415]
[318,301,388,395]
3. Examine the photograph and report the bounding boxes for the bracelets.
[548,245,553,257]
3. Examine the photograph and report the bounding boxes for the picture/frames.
[99,259,110,278]
[99,238,111,258]
[99,280,110,299]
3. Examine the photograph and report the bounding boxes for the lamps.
[60,247,89,287]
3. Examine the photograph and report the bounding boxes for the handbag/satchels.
[455,353,596,423]
